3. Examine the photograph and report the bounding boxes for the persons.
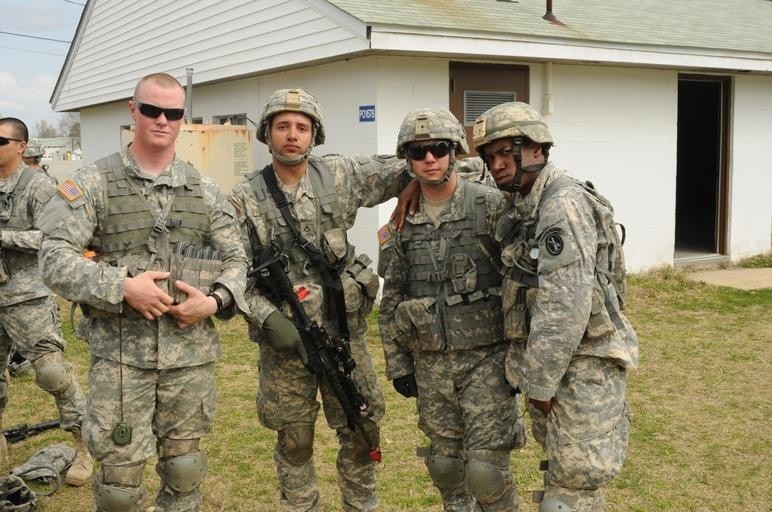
[0,118,96,486]
[378,107,527,512]
[390,100,640,512]
[226,88,490,512]
[36,72,252,512]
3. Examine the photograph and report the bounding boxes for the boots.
[0,416,9,462]
[65,426,95,486]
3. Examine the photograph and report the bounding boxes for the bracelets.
[207,292,223,313]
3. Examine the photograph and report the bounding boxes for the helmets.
[397,108,469,159]
[256,89,325,147]
[23,140,45,159]
[472,101,554,152]
[1,475,38,512]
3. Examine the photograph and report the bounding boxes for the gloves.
[393,373,418,398]
[262,311,310,366]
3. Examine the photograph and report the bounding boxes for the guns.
[2,422,61,443]
[247,258,381,462]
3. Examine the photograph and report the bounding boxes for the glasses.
[404,140,450,161]
[133,99,185,121]
[0,136,23,146]
